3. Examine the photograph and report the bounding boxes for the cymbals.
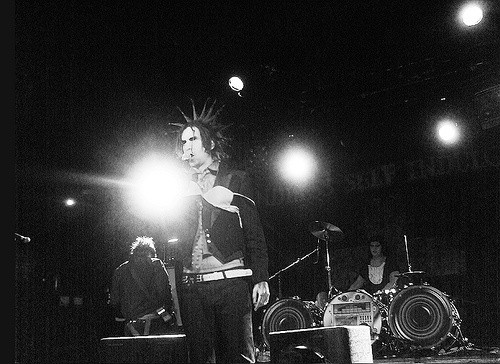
[394,271,426,278]
[308,220,344,244]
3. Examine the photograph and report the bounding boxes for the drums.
[259,296,324,351]
[322,289,387,351]
[372,287,401,306]
[387,284,461,354]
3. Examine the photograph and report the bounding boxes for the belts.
[136,313,160,320]
[181,269,252,283]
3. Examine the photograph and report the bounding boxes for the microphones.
[317,239,319,261]
[182,153,191,166]
[14,233,30,243]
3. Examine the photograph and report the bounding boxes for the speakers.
[99,334,188,364]
[269,325,373,364]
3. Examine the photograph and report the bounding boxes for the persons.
[112,237,182,364]
[160,121,271,364]
[347,236,400,297]
